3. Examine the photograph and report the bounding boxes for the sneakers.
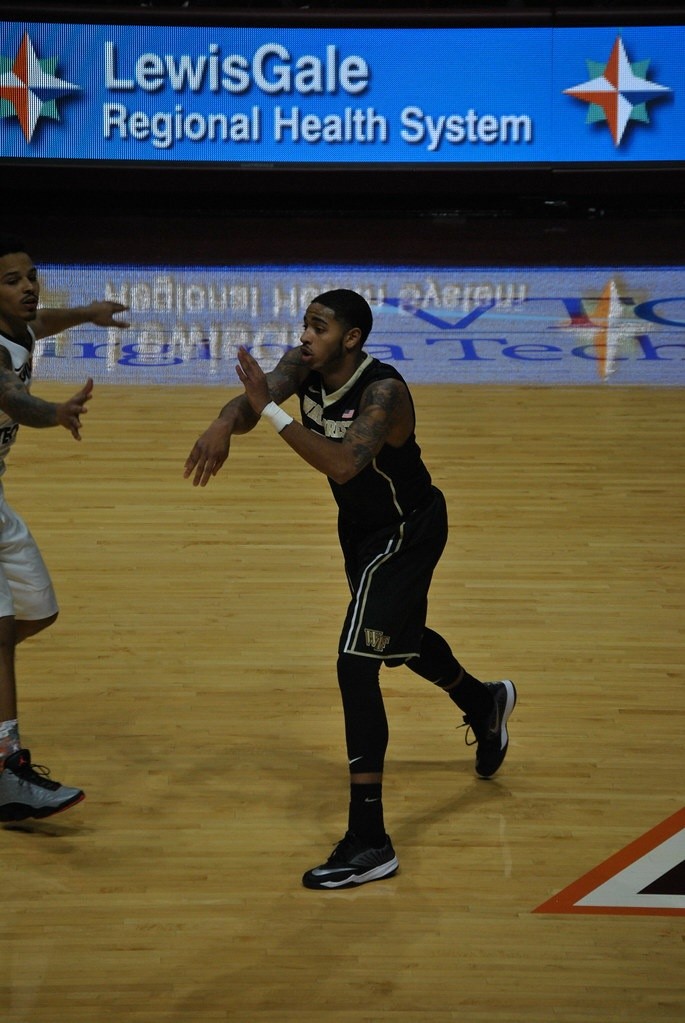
[303,826,399,892]
[0,749,85,822]
[456,678,518,783]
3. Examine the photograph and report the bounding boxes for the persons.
[0,246,133,822]
[181,289,518,892]
